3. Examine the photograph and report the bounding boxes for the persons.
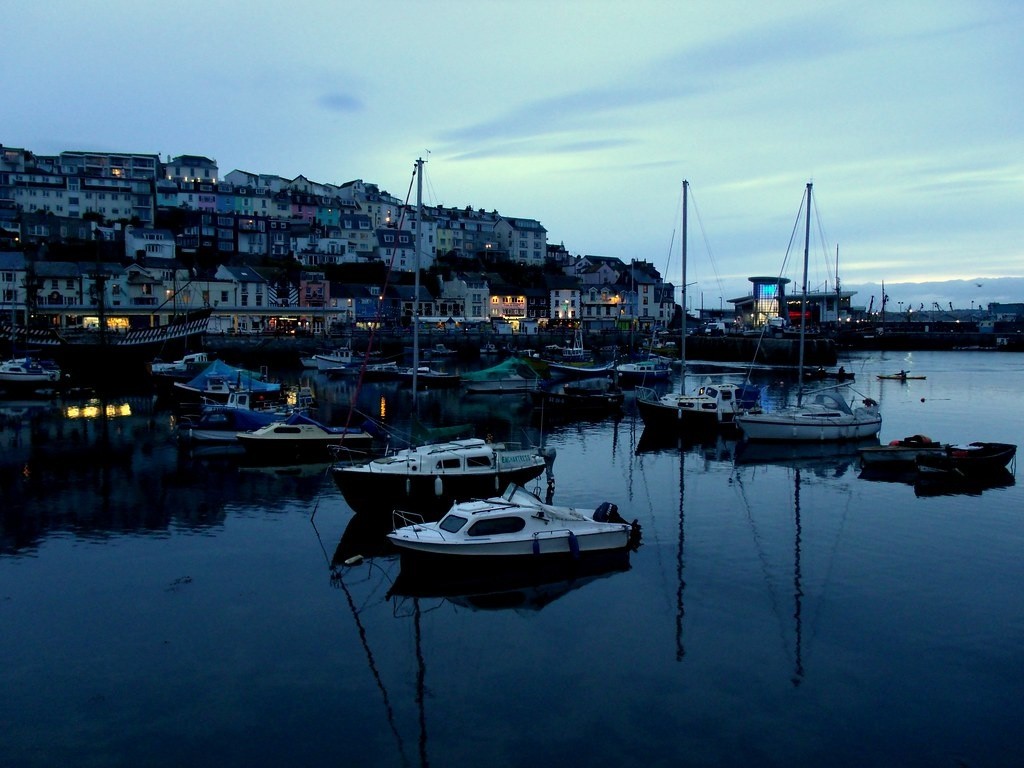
[901,370,905,376]
[839,366,844,372]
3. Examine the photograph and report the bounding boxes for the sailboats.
[635,178,763,428]
[326,158,557,493]
[732,182,882,440]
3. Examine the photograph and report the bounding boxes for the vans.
[705,323,725,335]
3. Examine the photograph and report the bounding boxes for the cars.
[855,442,1018,467]
[300,353,317,366]
[173,390,318,441]
[480,343,498,353]
[234,411,381,449]
[431,344,459,355]
[545,345,562,349]
[876,375,926,379]
[547,362,616,376]
[0,362,61,381]
[316,346,367,369]
[1,357,35,365]
[171,359,285,394]
[399,367,460,382]
[150,351,207,375]
[511,350,536,354]
[385,482,634,557]
[543,329,594,362]
[358,361,398,377]
[461,358,543,393]
[616,360,674,379]
